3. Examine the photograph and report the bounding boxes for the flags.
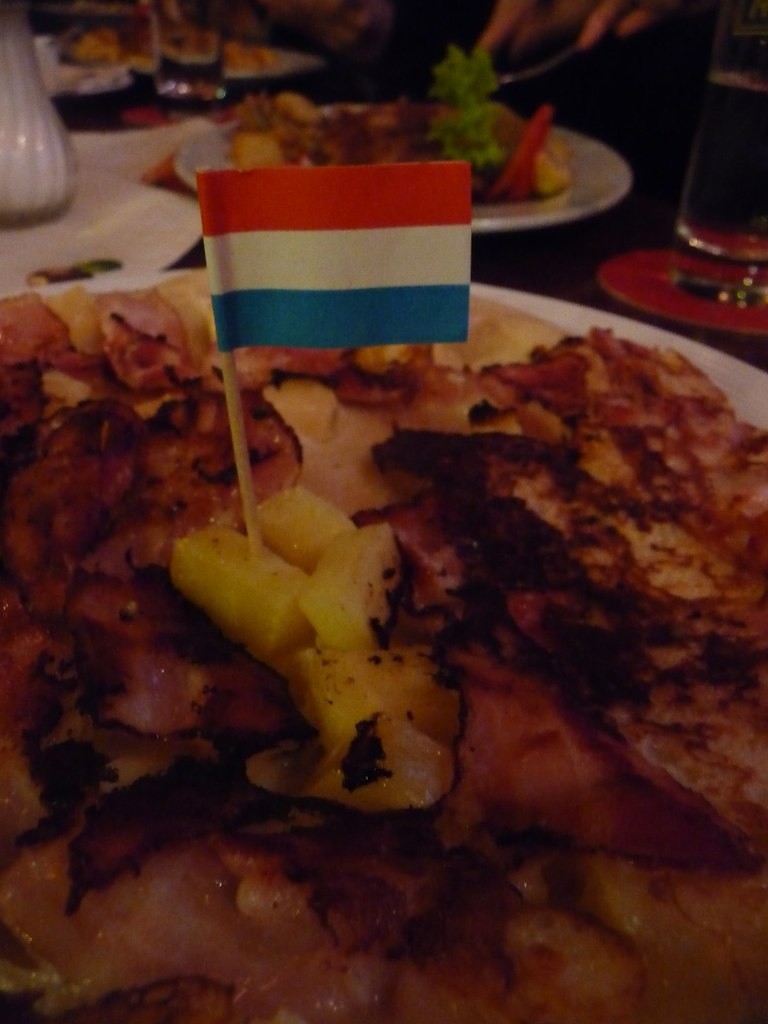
[196,160,471,353]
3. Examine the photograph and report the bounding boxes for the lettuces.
[424,45,510,168]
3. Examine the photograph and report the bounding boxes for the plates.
[173,104,632,232]
[0,173,204,294]
[0,269,768,1024]
[132,43,322,79]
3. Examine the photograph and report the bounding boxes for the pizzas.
[0,267,768,1024]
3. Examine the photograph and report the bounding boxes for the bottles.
[668,0,768,306]
[0,1,79,228]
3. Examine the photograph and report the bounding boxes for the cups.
[150,1,228,101]
